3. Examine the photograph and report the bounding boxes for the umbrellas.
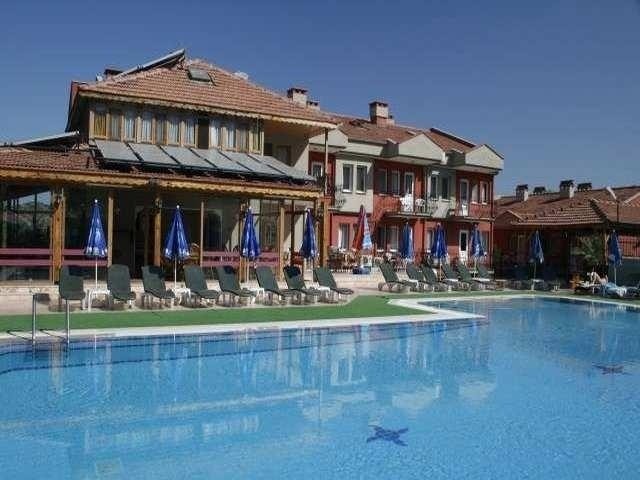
[296,328,315,391]
[466,223,489,273]
[430,221,448,282]
[351,205,376,265]
[526,297,542,343]
[431,323,449,365]
[82,199,110,300]
[162,204,191,295]
[85,335,110,403]
[236,206,263,289]
[526,231,547,279]
[297,207,321,286]
[237,331,258,399]
[401,219,417,274]
[604,230,624,286]
[168,334,189,403]
[396,323,412,373]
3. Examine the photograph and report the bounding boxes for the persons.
[589,272,609,287]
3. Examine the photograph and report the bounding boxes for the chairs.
[377,262,508,293]
[58,263,353,312]
